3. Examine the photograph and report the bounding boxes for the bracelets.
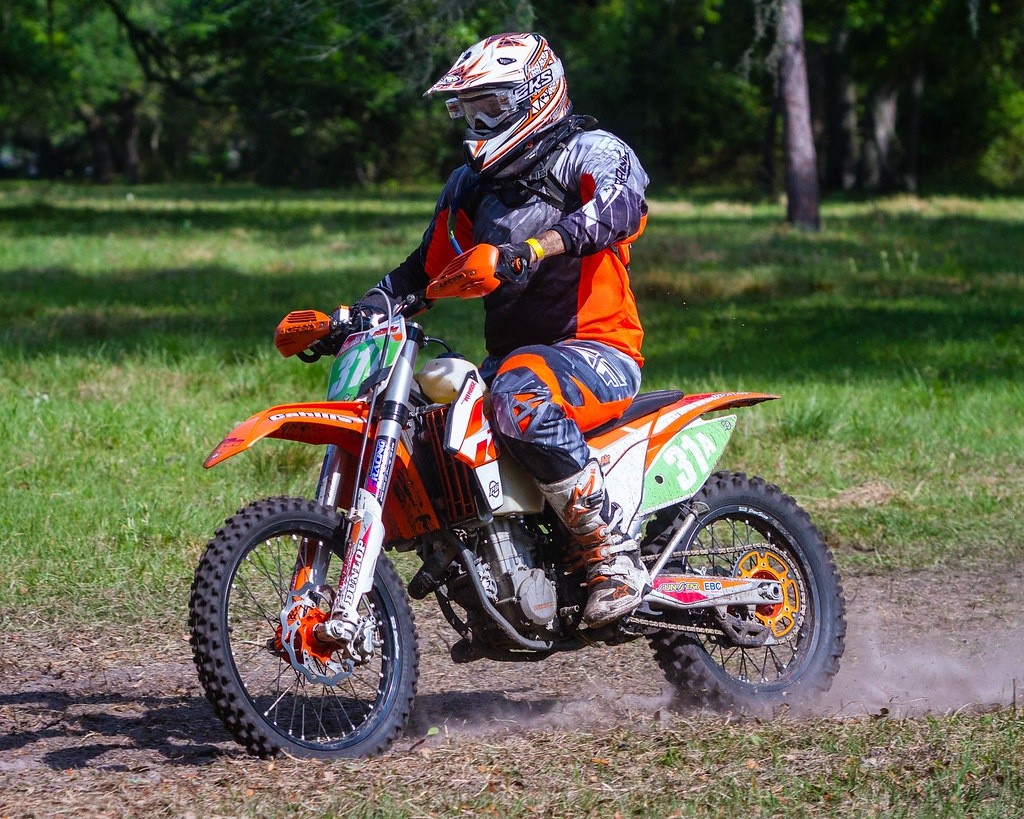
[524,238,545,262]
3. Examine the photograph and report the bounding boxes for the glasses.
[457,95,506,126]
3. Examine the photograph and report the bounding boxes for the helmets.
[422,32,572,175]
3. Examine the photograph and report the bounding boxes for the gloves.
[309,333,344,357]
[495,238,545,298]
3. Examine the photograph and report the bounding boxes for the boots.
[451,638,485,664]
[533,457,654,627]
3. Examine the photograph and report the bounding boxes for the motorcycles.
[187,243,848,763]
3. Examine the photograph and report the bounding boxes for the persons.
[312,34,653,663]
